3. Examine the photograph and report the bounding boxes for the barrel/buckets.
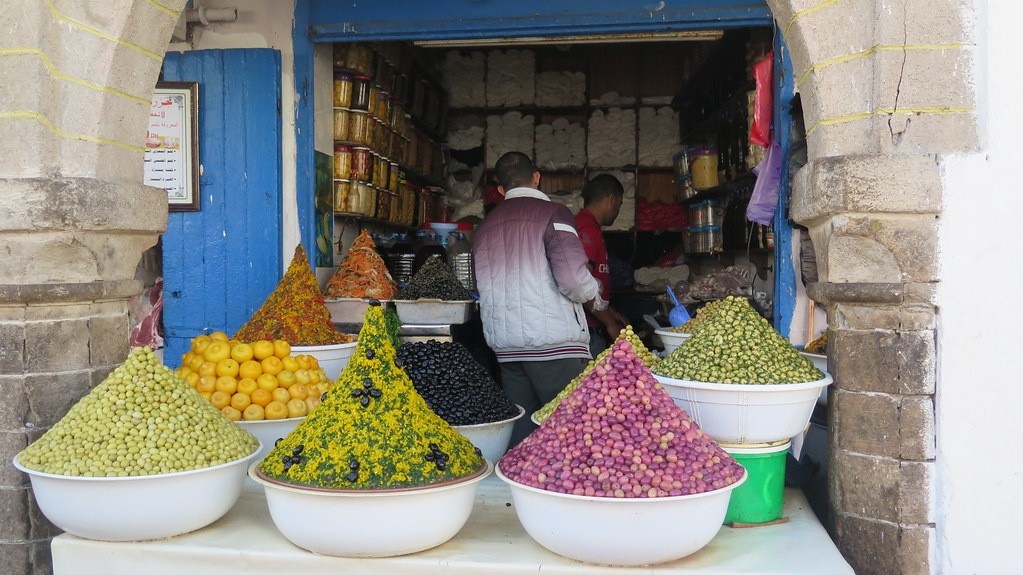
[688,147,719,192]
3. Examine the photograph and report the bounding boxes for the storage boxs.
[322,296,392,324]
[394,297,475,325]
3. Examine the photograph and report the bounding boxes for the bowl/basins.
[12,439,265,543]
[798,351,828,407]
[651,372,834,444]
[248,455,495,559]
[654,326,691,356]
[494,460,747,568]
[450,403,526,466]
[232,417,306,494]
[289,333,358,382]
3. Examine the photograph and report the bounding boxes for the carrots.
[171,330,336,420]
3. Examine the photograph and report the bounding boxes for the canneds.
[334,43,440,229]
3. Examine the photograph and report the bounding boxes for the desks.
[51,463,857,575]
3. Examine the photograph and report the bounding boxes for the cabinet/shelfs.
[332,43,793,260]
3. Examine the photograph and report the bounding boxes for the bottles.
[370,222,477,293]
[717,101,776,254]
[334,41,434,229]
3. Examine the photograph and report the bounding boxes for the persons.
[472,152,625,454]
[574,173,631,360]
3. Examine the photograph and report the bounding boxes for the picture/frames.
[142,81,200,211]
